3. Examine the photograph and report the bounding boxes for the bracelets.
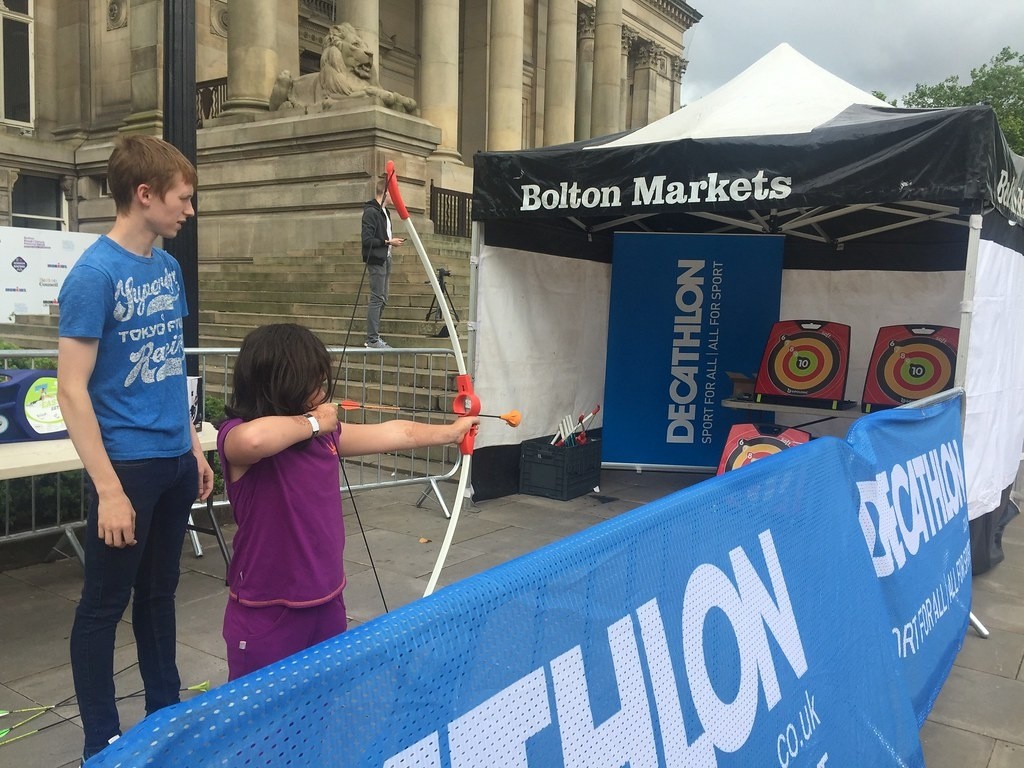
[303,412,320,438]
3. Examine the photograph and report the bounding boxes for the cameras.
[437,267,450,277]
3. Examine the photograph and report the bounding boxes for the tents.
[469,39,1024,576]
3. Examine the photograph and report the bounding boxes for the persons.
[361,176,404,351]
[218,322,480,692]
[51,134,216,768]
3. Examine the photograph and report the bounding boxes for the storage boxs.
[519,434,602,501]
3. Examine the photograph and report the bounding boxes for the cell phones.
[403,237,407,241]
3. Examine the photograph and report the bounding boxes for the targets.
[859,324,961,414]
[715,421,813,477]
[754,319,852,409]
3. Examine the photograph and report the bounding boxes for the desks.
[0,422,233,587]
[721,397,868,419]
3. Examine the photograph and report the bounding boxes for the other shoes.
[364,340,368,347]
[366,340,392,348]
[79,745,111,768]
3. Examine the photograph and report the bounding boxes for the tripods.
[425,277,460,323]
